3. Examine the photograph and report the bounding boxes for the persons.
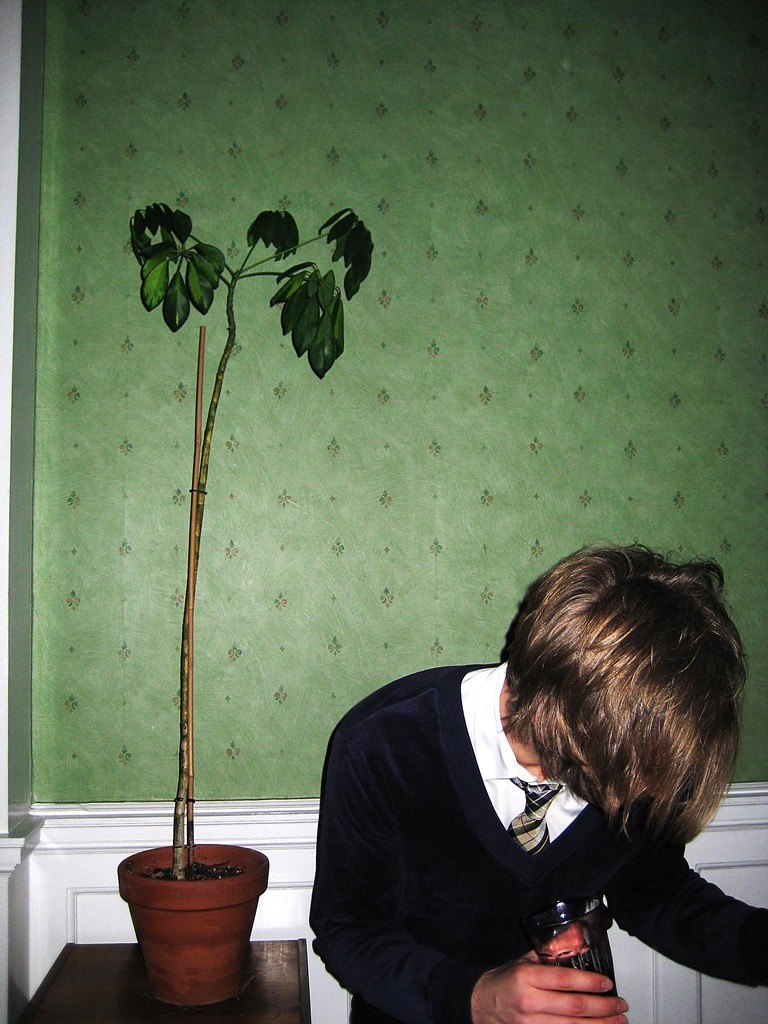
[311,546,768,1024]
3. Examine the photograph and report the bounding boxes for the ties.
[509,776,564,856]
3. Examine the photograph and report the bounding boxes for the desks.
[15,939,313,1024]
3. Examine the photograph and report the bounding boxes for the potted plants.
[117,202,375,1003]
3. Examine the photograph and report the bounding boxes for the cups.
[523,898,619,998]
